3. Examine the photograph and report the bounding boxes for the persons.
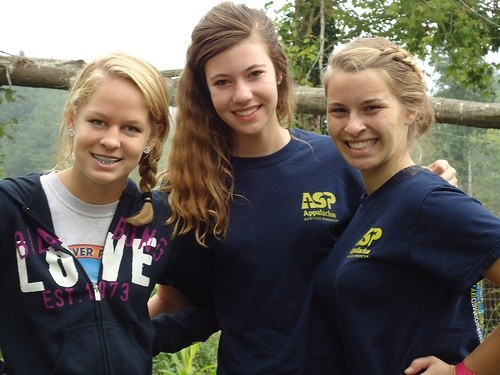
[264,34,500,375]
[146,4,458,375]
[0,54,169,375]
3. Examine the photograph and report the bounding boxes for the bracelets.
[454,361,476,375]
